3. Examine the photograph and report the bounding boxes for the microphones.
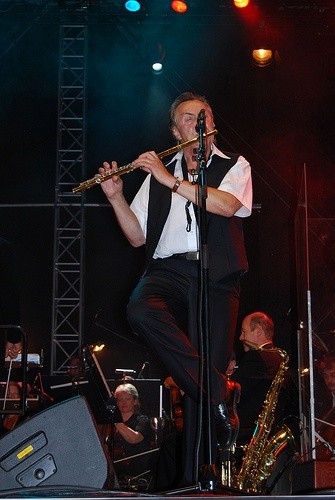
[195,108,207,135]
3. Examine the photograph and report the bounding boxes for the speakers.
[0,393,116,491]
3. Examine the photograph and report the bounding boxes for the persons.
[95,93,252,494]
[112,383,152,483]
[68,359,85,376]
[3,326,39,365]
[229,312,299,496]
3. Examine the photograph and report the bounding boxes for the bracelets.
[172,177,182,192]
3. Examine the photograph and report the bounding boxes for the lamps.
[246,32,274,68]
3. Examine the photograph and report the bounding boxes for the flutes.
[70,129,219,195]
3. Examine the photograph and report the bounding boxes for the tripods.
[156,137,261,496]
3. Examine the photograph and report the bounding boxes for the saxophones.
[233,339,296,495]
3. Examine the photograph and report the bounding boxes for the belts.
[168,251,200,261]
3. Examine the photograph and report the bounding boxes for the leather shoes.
[213,380,241,449]
